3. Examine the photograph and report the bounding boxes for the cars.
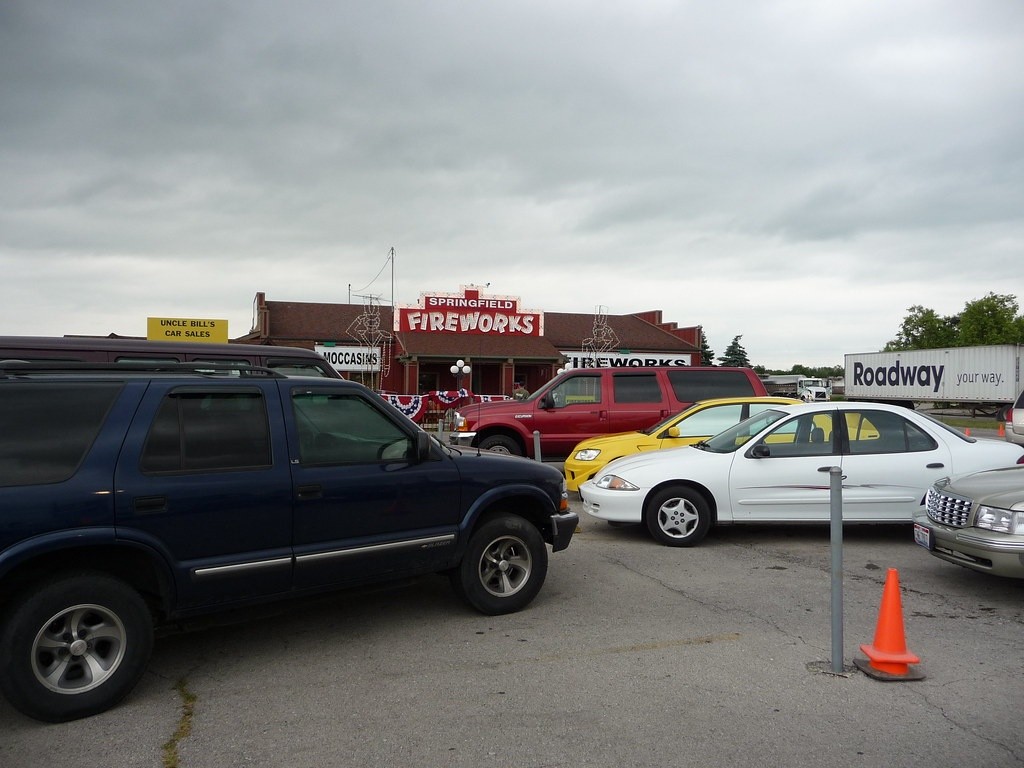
[563,396,880,492]
[576,401,1024,547]
[912,464,1024,579]
[1006,387,1024,448]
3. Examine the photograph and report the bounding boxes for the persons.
[513,381,529,400]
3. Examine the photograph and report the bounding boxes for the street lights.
[450,360,471,409]
[557,363,573,395]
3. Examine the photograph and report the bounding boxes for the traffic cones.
[860,567,921,663]
[965,428,970,436]
[853,659,926,682]
[998,424,1006,437]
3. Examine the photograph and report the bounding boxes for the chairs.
[808,428,825,451]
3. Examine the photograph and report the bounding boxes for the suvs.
[449,365,769,459]
[1,358,580,725]
[0,336,407,440]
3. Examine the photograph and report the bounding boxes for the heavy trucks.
[757,374,832,402]
